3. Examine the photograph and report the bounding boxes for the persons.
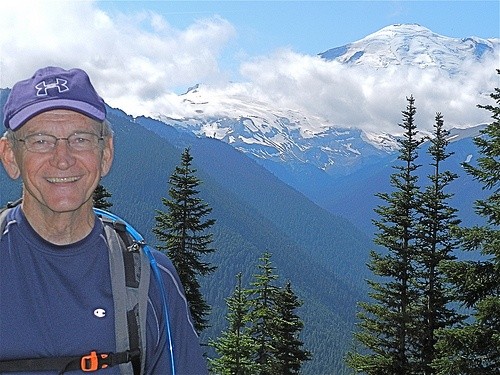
[0,67,209,375]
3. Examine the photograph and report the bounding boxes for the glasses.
[13,132,104,152]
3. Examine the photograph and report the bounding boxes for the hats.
[3,66,107,131]
